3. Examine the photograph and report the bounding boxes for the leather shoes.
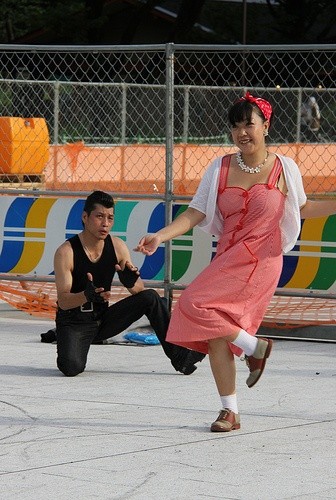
[210,408,240,432]
[246,337,273,389]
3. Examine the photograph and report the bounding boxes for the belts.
[65,301,109,312]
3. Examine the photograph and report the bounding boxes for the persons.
[133,94,307,431]
[54,191,207,377]
[307,97,321,143]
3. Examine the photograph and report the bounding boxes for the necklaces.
[237,148,269,174]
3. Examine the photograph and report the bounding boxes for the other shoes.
[177,349,206,376]
